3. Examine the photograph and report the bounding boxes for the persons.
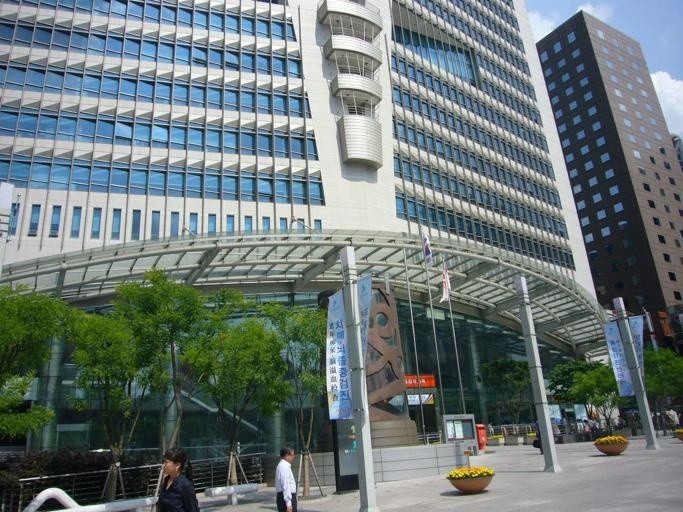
[534,404,683,454]
[488,421,494,437]
[512,419,519,435]
[275,445,297,512]
[157,447,200,512]
[500,422,508,437]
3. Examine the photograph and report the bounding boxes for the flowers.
[528,432,536,437]
[594,435,627,444]
[674,428,683,435]
[447,466,494,477]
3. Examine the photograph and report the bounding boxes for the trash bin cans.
[475,423,487,450]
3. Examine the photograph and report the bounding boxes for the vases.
[446,476,493,493]
[594,443,627,454]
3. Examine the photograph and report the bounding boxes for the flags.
[438,261,453,304]
[421,230,432,265]
